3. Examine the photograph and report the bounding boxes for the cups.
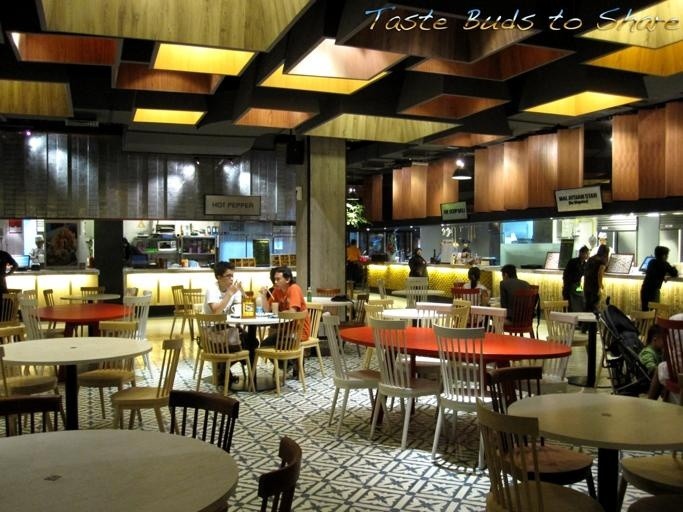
[230,304,241,316]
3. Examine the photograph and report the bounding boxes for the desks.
[507,393,682,512]
[339,327,572,426]
[61,293,121,303]
[549,312,631,388]
[28,304,134,336]
[382,308,446,327]
[0,428,239,511]
[200,314,293,392]
[0,337,152,430]
[299,297,366,356]
[392,290,445,302]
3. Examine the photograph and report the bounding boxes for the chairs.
[127,288,138,296]
[19,300,43,339]
[180,289,202,341]
[515,311,578,395]
[364,306,383,324]
[43,289,56,329]
[299,303,326,382]
[451,288,481,328]
[80,287,106,304]
[431,323,500,470]
[504,285,539,338]
[630,308,655,347]
[112,338,183,435]
[123,290,154,379]
[454,283,467,288]
[656,316,683,403]
[342,294,369,327]
[77,320,142,419]
[462,305,508,370]
[485,366,598,499]
[406,277,428,309]
[316,287,340,316]
[170,285,192,339]
[647,301,670,320]
[0,327,69,426]
[322,311,381,441]
[195,314,257,396]
[475,397,604,511]
[626,494,682,512]
[0,294,25,326]
[0,395,60,437]
[258,437,302,512]
[377,279,387,300]
[0,347,66,431]
[543,300,589,365]
[169,390,239,453]
[5,289,22,294]
[618,450,682,511]
[248,312,306,398]
[451,301,470,328]
[368,315,442,450]
[368,300,394,309]
[400,302,452,367]
[24,290,37,300]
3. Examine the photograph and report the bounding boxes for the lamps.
[346,168,359,201]
[451,149,473,181]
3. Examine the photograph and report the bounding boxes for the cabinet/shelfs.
[135,237,180,268]
[180,235,216,267]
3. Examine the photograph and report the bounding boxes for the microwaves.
[156,240,177,252]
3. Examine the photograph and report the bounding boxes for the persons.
[500,264,530,326]
[1,246,19,318]
[409,248,426,302]
[347,238,367,280]
[563,245,589,311]
[464,266,489,324]
[29,235,45,264]
[204,262,259,386]
[641,245,679,328]
[647,309,683,402]
[631,325,669,394]
[582,245,610,334]
[260,266,310,376]
[122,239,144,266]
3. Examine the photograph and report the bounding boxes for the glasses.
[219,274,233,279]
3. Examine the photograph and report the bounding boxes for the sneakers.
[292,361,305,381]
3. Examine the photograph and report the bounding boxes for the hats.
[35,237,44,241]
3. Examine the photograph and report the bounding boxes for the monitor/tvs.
[11,255,30,267]
